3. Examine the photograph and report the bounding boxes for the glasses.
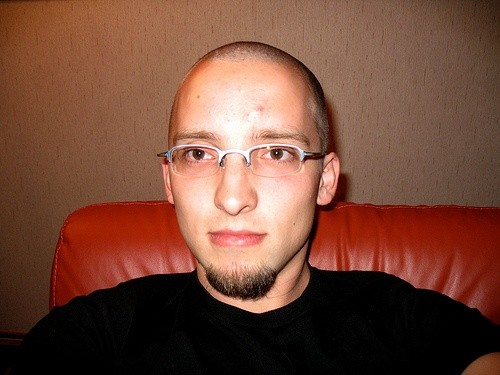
[157,144,328,178]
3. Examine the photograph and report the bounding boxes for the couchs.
[49,201,499,333]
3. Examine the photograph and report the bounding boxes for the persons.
[0,41,500,375]
